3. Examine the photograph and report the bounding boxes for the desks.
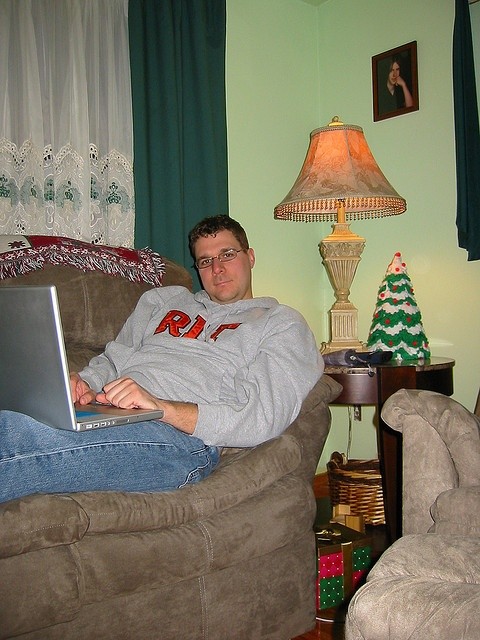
[318,355,455,540]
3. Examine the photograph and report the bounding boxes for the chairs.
[0,234,343,639]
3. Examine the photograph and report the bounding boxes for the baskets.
[326,451,386,527]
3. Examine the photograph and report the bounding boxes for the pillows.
[300,374,344,412]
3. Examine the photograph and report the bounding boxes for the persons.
[378,55,414,114]
[0,215,326,503]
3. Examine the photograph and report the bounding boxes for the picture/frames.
[372,40,419,122]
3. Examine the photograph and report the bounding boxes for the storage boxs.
[314,522,373,612]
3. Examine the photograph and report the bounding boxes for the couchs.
[346,389,479,640]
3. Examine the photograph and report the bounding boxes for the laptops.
[0,286,164,433]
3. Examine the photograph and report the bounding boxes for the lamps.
[274,116,407,357]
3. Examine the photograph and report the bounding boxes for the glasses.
[194,248,244,269]
